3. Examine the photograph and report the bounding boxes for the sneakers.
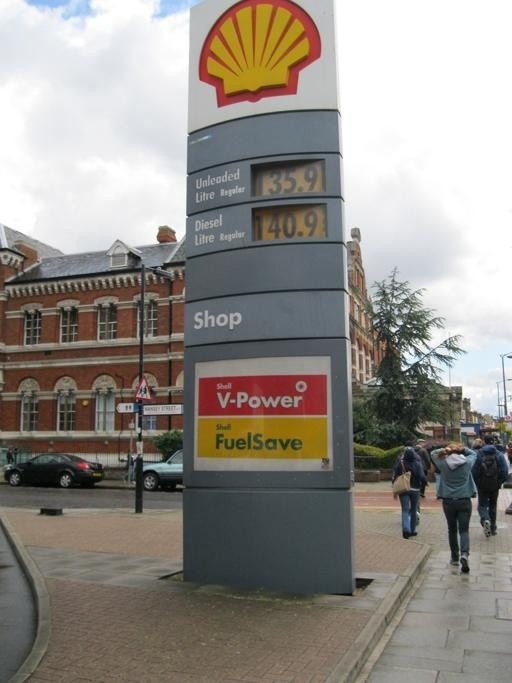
[460,555,470,572]
[483,519,492,537]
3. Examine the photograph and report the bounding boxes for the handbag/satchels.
[392,471,412,495]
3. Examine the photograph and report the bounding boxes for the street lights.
[499,351,512,417]
[499,394,512,417]
[496,378,512,418]
[133,263,176,513]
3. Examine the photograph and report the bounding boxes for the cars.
[4,452,105,490]
[142,448,183,491]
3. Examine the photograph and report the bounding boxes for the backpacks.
[478,449,500,489]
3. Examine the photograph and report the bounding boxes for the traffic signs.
[115,402,138,413]
[142,404,183,416]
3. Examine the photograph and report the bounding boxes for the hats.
[485,435,495,443]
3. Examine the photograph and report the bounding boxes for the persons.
[429,441,478,572]
[470,438,484,450]
[413,438,432,497]
[508,439,512,464]
[391,446,429,539]
[433,468,443,499]
[122,448,137,484]
[471,435,508,537]
[494,433,505,454]
